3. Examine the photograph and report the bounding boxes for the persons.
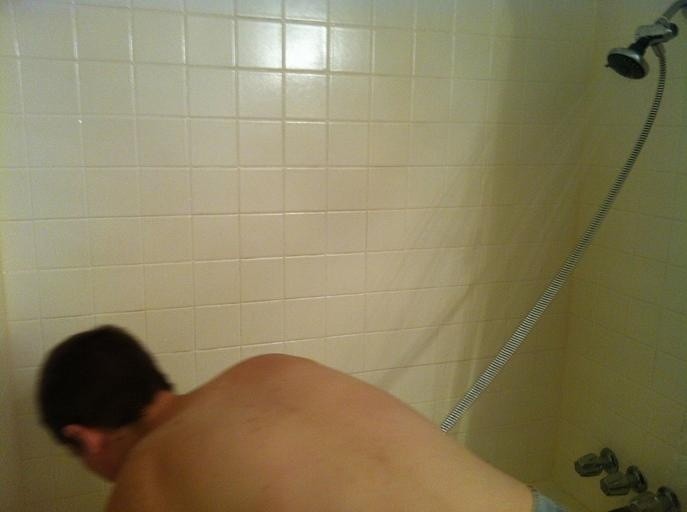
[39,326,561,512]
